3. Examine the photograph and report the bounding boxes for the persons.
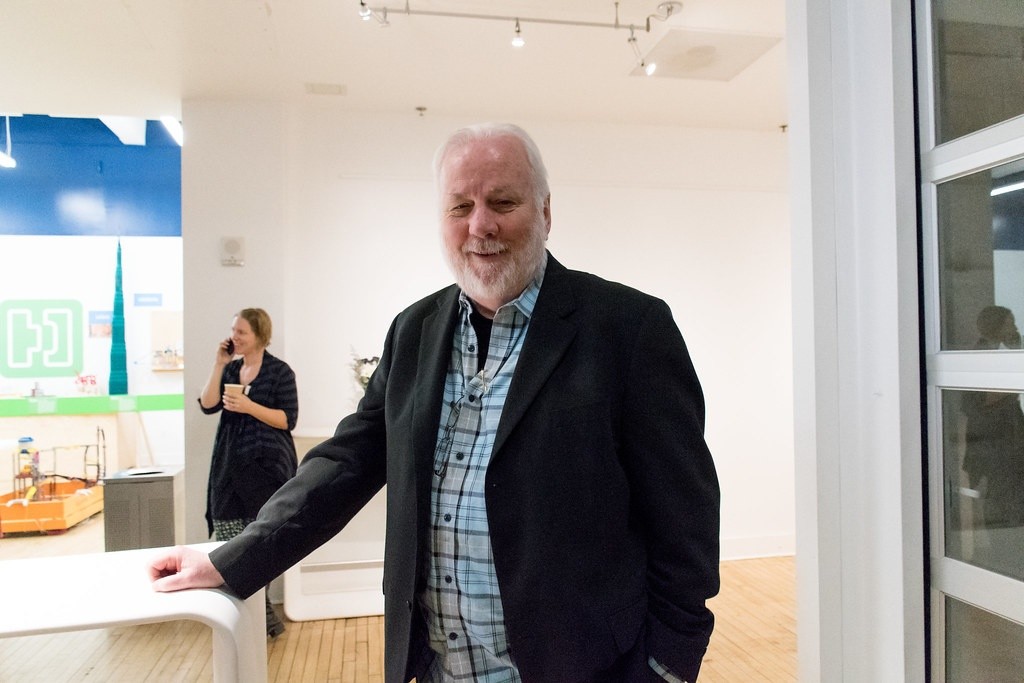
[960,307,1024,566]
[195,306,301,638]
[149,122,723,683]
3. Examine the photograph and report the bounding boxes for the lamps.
[510,19,525,50]
[628,29,656,76]
[359,2,387,28]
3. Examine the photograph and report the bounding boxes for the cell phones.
[226,339,234,354]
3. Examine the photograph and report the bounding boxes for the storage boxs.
[103,468,186,551]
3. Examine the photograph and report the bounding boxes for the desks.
[1,540,267,683]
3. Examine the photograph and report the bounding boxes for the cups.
[224,383,244,394]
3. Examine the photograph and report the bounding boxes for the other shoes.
[266,596,285,637]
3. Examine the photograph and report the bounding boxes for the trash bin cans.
[103,464,185,552]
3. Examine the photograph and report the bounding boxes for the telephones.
[225,338,235,355]
